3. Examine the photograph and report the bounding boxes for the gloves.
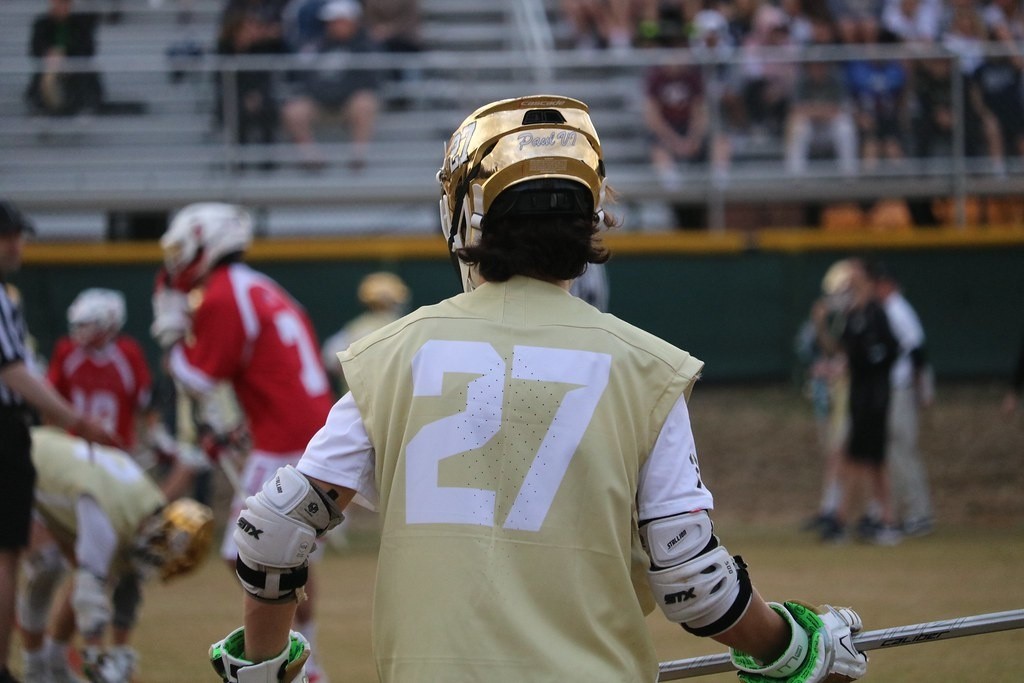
[729,590,869,683]
[208,623,311,683]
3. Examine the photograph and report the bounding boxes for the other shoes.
[867,514,935,549]
[851,513,885,545]
[799,513,849,543]
[23,666,88,682]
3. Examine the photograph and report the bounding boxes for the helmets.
[820,257,859,298]
[357,270,411,307]
[435,92,608,291]
[66,287,126,351]
[136,495,216,581]
[158,202,252,291]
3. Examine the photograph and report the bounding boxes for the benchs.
[0,0,1024,241]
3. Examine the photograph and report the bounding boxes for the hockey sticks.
[660,604,1024,682]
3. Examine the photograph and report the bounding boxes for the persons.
[318,272,408,396]
[152,202,336,682]
[28,1,102,114]
[23,426,212,682]
[48,288,150,457]
[164,0,428,175]
[1,200,122,682]
[800,260,937,541]
[208,95,868,683]
[154,270,256,518]
[551,1,1024,233]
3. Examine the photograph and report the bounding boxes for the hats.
[0,197,38,242]
